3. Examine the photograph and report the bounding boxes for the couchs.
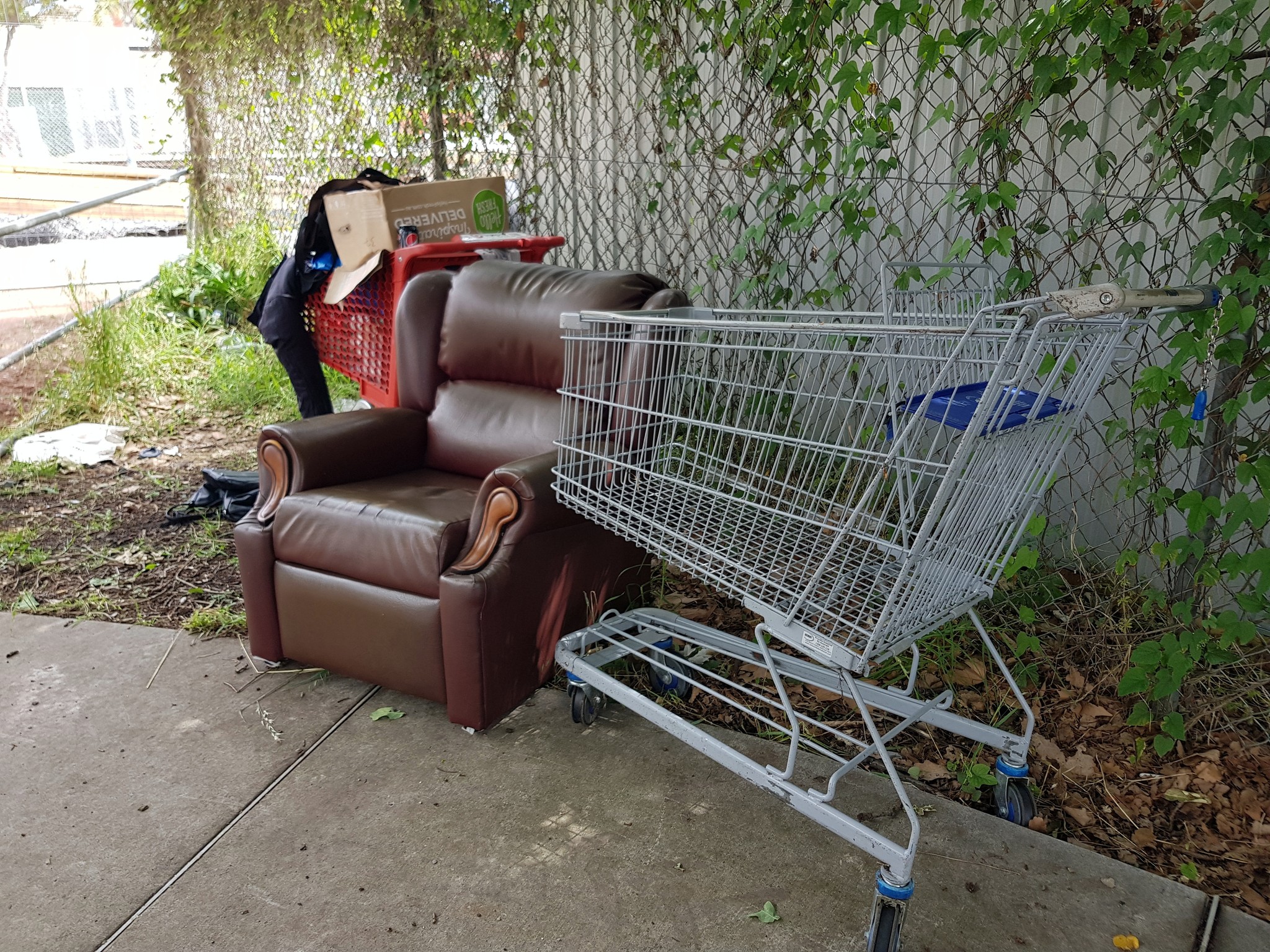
[232,260,698,730]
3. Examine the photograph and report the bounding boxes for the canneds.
[398,224,419,250]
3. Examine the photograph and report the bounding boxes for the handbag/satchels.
[165,466,261,522]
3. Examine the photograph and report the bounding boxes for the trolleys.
[543,258,1224,951]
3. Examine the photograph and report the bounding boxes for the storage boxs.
[322,174,508,305]
